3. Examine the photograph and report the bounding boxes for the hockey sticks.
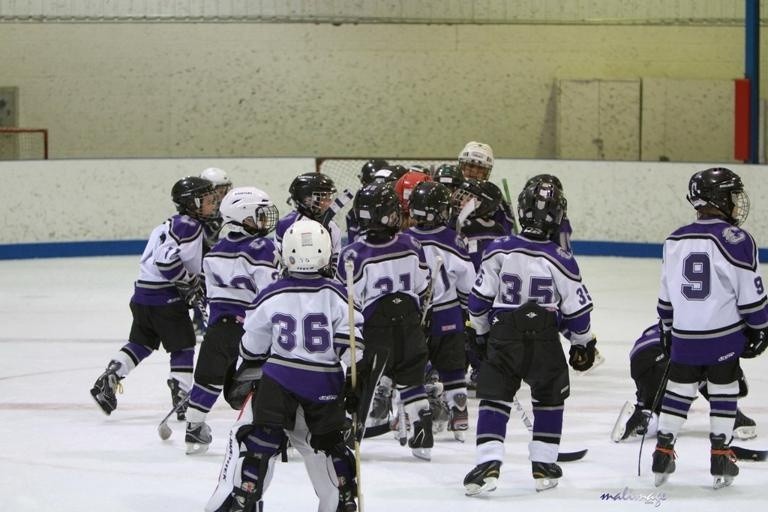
[158,391,188,439]
[637,357,671,477]
[579,347,605,379]
[515,397,588,462]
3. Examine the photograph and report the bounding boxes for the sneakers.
[462,459,502,485]
[532,462,562,480]
[733,404,756,430]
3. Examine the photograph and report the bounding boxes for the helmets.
[687,167,750,226]
[169,166,231,203]
[516,171,566,236]
[351,158,503,231]
[456,141,494,171]
[218,186,279,235]
[280,219,334,273]
[288,171,338,215]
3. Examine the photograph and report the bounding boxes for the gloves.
[474,330,490,356]
[177,273,205,306]
[342,357,368,414]
[659,324,674,359]
[224,356,262,409]
[569,340,597,371]
[743,322,766,360]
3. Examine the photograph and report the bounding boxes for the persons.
[651,168,768,475]
[619,316,768,440]
[94,176,219,413]
[193,166,234,337]
[274,172,342,280]
[215,219,365,512]
[184,188,280,443]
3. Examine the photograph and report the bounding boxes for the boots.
[709,432,739,475]
[653,430,676,475]
[212,486,267,511]
[447,404,468,431]
[166,378,193,418]
[331,486,358,511]
[371,384,391,418]
[622,404,654,440]
[425,383,449,421]
[185,423,213,445]
[89,361,125,414]
[407,409,434,449]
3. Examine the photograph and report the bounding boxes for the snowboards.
[352,345,390,449]
